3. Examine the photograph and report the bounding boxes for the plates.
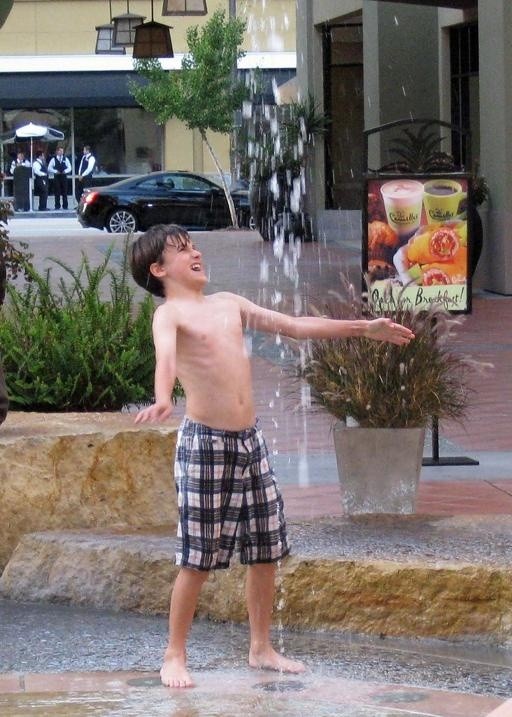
[393,220,467,286]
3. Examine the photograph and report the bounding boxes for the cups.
[380,178,467,238]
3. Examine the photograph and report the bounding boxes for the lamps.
[88,1,208,60]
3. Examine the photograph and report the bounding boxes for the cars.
[78,170,256,233]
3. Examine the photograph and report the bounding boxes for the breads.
[368,221,402,280]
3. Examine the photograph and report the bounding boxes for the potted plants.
[270,269,494,518]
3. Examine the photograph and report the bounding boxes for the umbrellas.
[0,120,65,166]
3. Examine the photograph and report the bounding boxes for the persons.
[32,151,50,211]
[75,145,96,203]
[127,224,416,688]
[47,145,72,210]
[10,151,31,174]
